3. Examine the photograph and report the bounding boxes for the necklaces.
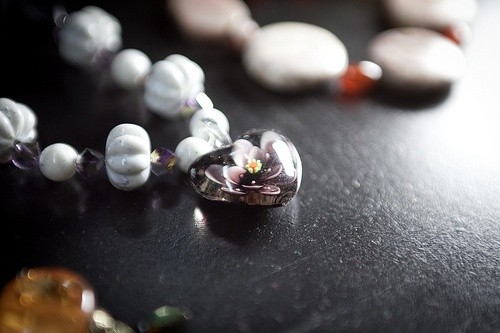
[1,0,307,209]
[167,0,483,94]
